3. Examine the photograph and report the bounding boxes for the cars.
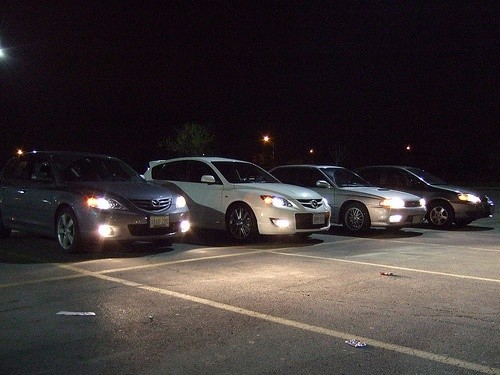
[0,151,192,254]
[259,164,429,235]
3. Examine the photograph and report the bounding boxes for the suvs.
[352,166,494,228]
[143,156,333,241]
